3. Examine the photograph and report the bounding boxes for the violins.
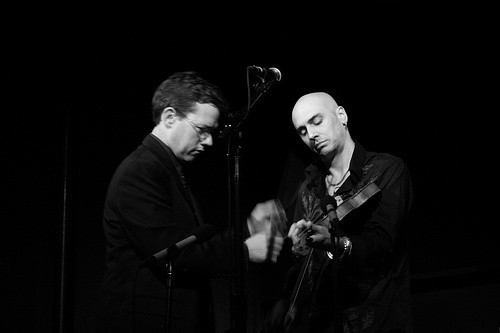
[289,180,384,258]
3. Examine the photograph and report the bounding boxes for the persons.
[284,92,414,333]
[103,73,283,333]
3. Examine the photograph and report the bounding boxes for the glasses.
[164,105,218,141]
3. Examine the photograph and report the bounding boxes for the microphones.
[249,64,282,81]
[135,223,216,271]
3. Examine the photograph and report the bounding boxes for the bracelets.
[327,237,352,263]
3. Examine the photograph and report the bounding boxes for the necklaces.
[327,169,349,193]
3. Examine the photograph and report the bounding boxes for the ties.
[179,171,193,210]
